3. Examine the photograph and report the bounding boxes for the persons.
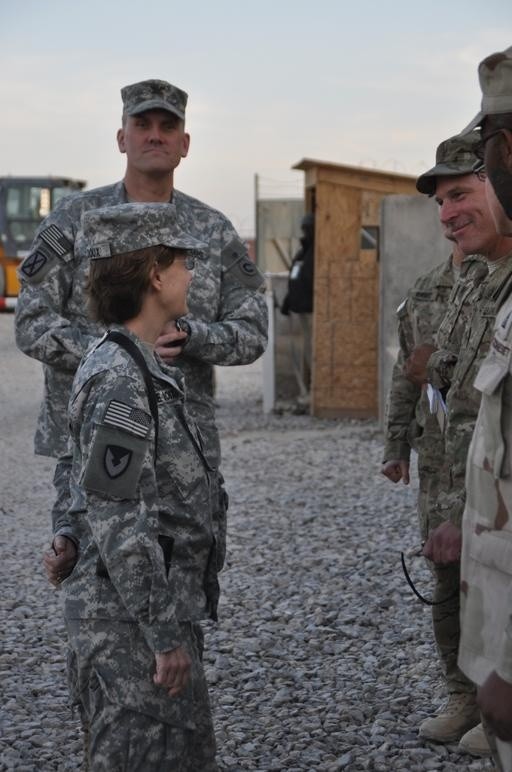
[44,197,230,772]
[452,41,512,771]
[16,78,272,626]
[378,202,473,539]
[414,107,500,761]
[280,212,314,413]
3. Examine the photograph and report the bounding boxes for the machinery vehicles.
[0,178,86,311]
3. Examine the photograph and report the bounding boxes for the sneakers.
[420,694,493,758]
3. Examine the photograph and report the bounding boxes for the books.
[426,382,440,414]
[437,383,449,435]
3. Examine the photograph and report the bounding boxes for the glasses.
[174,256,197,271]
[472,130,503,181]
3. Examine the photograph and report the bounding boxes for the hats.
[416,129,480,197]
[460,46,512,137]
[120,79,188,118]
[80,202,208,260]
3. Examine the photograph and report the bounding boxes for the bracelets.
[174,317,189,346]
